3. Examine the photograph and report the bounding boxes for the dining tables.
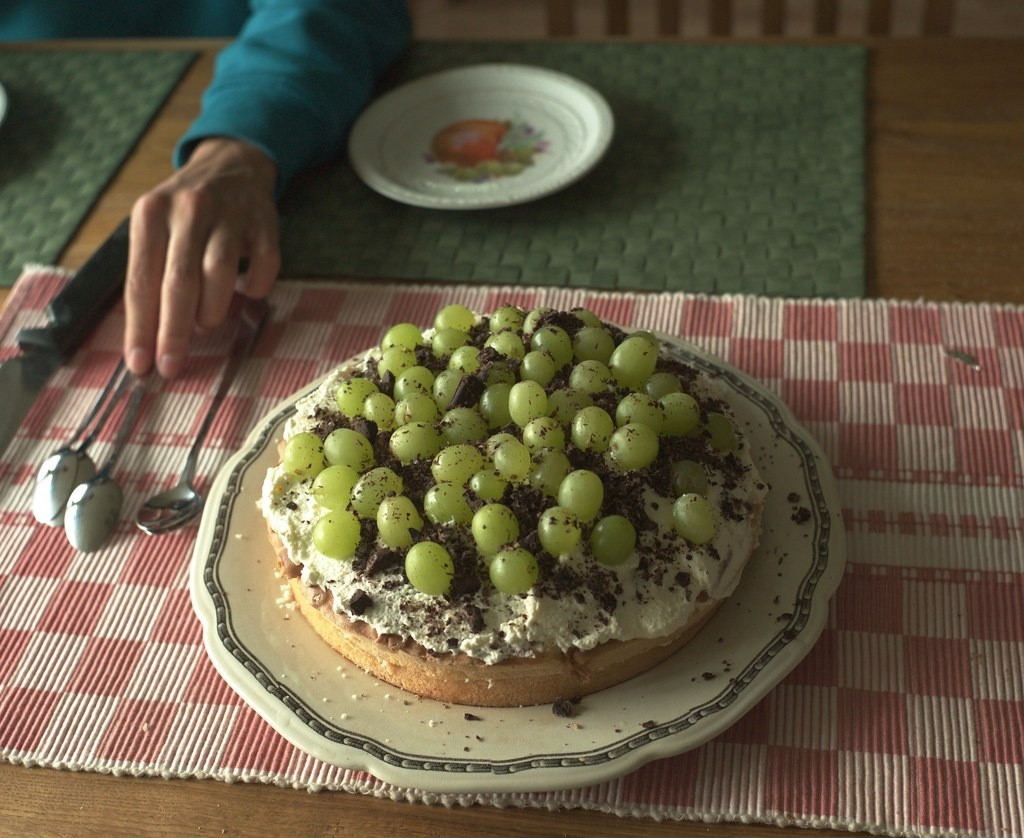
[0,36,1024,838]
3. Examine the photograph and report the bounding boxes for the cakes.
[258,306,764,701]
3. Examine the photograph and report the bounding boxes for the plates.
[346,64,616,208]
[191,309,850,789]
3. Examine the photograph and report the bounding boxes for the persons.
[0,0,416,379]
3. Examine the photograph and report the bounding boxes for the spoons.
[135,318,261,540]
[31,358,124,523]
[43,368,131,529]
[66,365,152,553]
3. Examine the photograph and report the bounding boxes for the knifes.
[0,216,135,459]
